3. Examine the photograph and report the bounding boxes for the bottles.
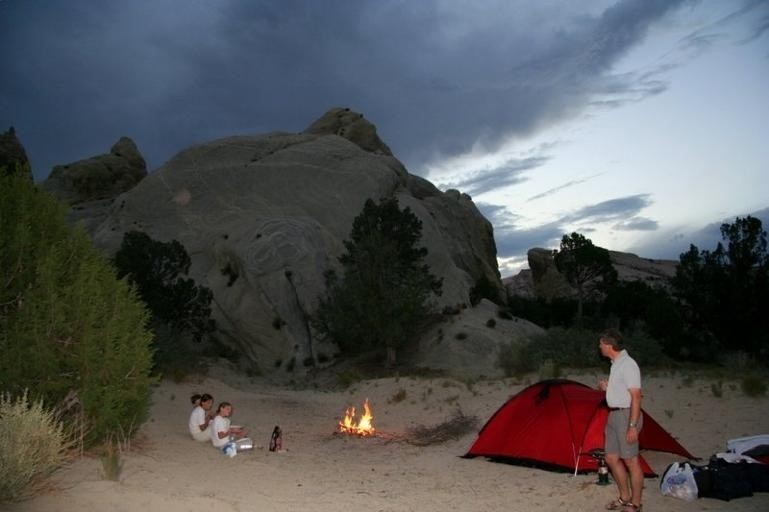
[598,457,608,484]
[230,437,237,455]
[271,425,282,450]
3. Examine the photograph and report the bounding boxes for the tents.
[459,377,696,478]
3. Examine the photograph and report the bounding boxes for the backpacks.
[660,444,769,502]
[269,425,282,451]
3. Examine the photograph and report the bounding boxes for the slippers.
[605,496,643,512]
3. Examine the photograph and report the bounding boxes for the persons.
[187,394,251,449]
[598,328,645,512]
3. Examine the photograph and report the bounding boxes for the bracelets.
[628,422,637,428]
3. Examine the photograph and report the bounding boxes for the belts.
[610,408,624,411]
